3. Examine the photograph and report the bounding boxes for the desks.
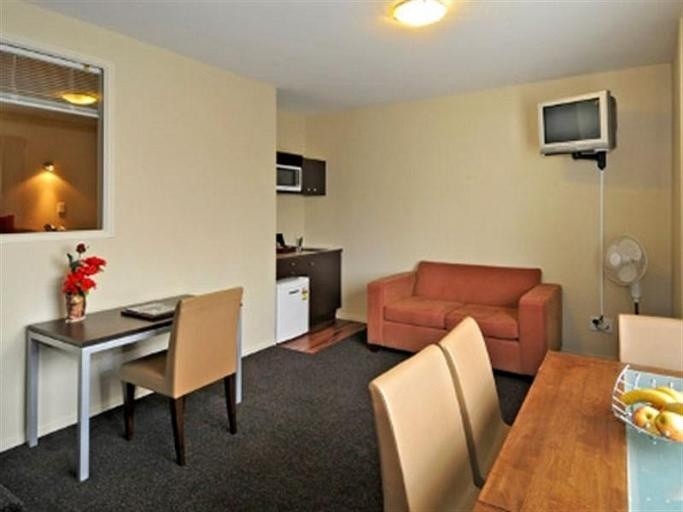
[26,295,244,483]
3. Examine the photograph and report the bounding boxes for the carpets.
[279,319,367,354]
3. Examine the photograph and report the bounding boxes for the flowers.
[64,244,105,294]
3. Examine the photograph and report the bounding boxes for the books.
[121,302,175,322]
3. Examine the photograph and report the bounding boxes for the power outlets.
[589,317,604,330]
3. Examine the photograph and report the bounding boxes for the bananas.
[621,389,674,412]
[660,401,682,412]
[655,385,682,405]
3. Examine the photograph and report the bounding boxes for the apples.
[633,407,657,429]
[659,411,682,441]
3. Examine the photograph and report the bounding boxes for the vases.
[65,294,85,323]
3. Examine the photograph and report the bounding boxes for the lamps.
[60,90,99,105]
[391,0,448,30]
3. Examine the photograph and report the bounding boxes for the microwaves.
[276,165,302,192]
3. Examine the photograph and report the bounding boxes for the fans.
[604,234,649,314]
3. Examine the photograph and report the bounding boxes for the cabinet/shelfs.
[277,252,341,333]
[302,159,325,195]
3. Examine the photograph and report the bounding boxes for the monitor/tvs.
[537,89,616,154]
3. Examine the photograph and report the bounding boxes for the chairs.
[619,313,683,372]
[120,287,243,466]
[368,344,481,512]
[439,316,512,486]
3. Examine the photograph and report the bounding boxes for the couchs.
[366,262,562,377]
